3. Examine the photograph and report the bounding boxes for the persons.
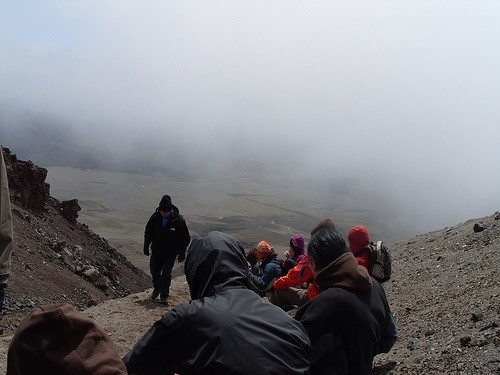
[268,218,336,308]
[284,235,306,264]
[292,228,397,375]
[121,230,313,375]
[143,195,191,304]
[347,225,392,284]
[6,303,129,375]
[248,240,282,298]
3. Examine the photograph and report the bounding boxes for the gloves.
[143,244,149,256]
[178,250,185,262]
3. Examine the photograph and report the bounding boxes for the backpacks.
[267,258,297,274]
[357,242,391,283]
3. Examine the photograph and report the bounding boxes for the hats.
[159,195,172,211]
[311,219,336,235]
[256,240,272,253]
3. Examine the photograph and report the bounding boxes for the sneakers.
[151,288,159,298]
[160,298,168,305]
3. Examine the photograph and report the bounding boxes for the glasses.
[161,209,171,212]
